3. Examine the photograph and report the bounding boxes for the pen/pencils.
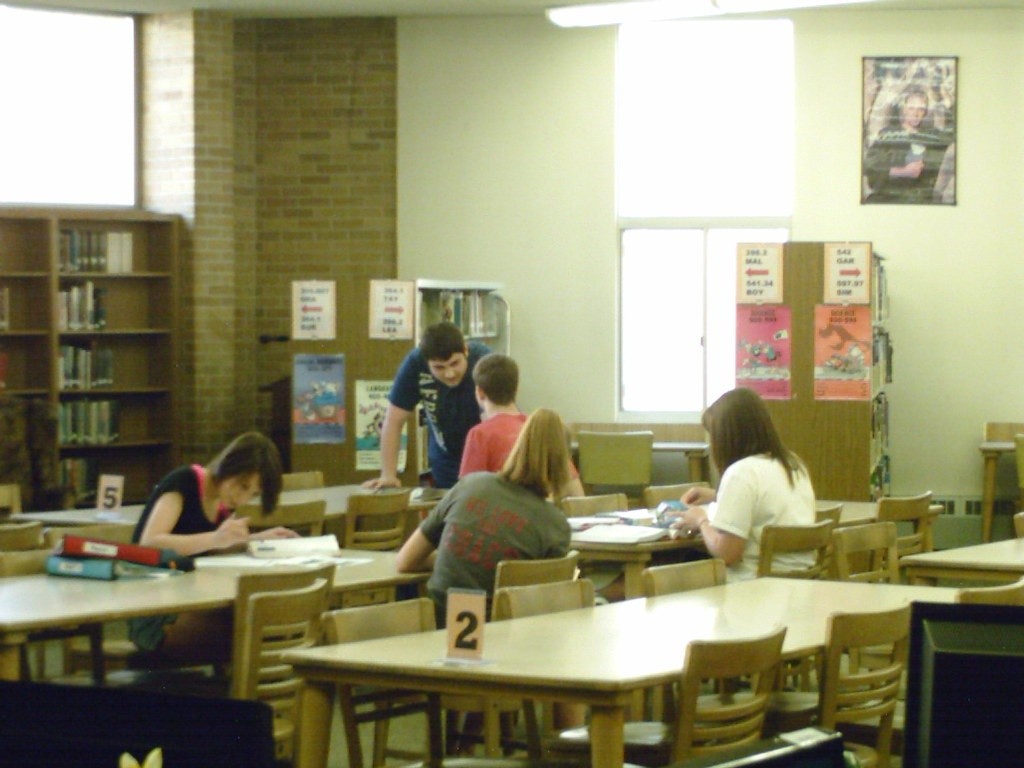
[221,502,228,518]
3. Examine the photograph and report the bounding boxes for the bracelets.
[698,519,709,532]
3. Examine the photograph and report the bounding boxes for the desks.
[977,442,1018,544]
[1,549,436,688]
[566,498,944,601]
[900,536,1023,588]
[280,577,957,768]
[571,442,709,481]
[9,485,451,527]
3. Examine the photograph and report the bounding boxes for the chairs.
[0,423,1024,768]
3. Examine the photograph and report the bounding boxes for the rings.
[688,531,693,535]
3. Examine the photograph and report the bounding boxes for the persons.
[127,432,300,666]
[359,322,492,489]
[863,58,955,203]
[667,386,817,581]
[457,353,585,498]
[395,404,571,625]
[300,381,342,421]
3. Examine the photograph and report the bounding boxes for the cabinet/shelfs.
[736,241,888,576]
[290,276,509,552]
[0,204,181,514]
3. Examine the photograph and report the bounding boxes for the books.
[439,291,486,333]
[249,535,339,558]
[0,287,9,328]
[0,352,10,389]
[871,261,893,501]
[59,228,133,499]
[47,534,184,580]
[566,508,688,544]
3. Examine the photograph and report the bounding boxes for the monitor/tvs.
[903,597,1024,768]
